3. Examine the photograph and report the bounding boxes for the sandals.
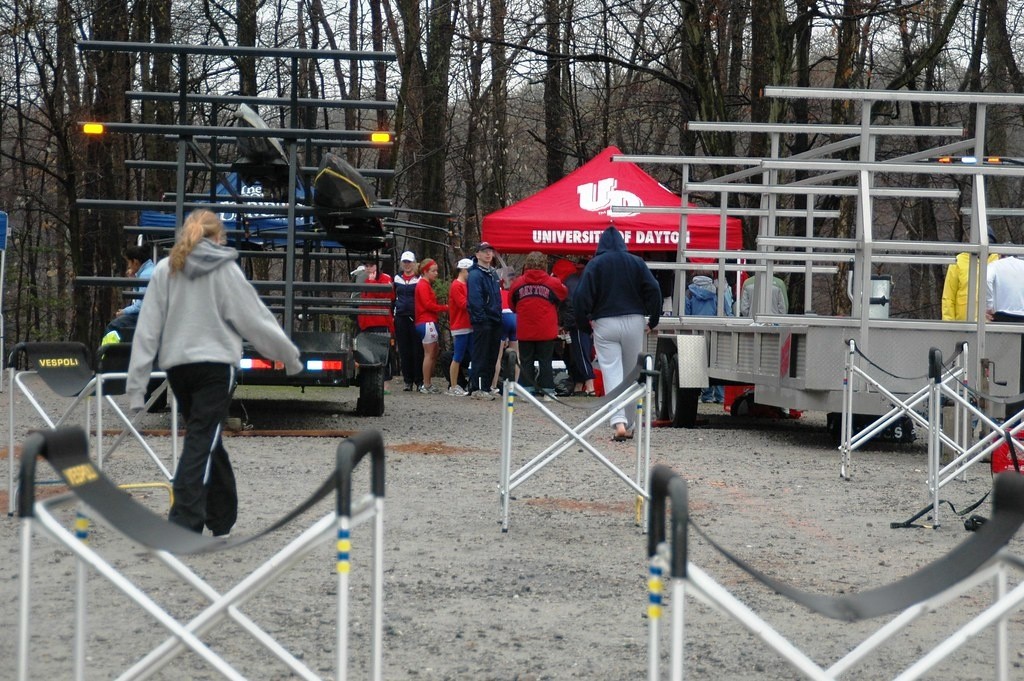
[579,389,596,396]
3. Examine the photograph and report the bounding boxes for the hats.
[457,259,474,268]
[401,251,414,262]
[475,241,494,252]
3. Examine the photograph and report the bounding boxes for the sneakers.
[471,390,495,400]
[420,384,441,393]
[449,385,469,396]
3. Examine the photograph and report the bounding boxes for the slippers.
[611,434,626,441]
[626,436,633,439]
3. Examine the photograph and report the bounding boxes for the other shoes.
[403,383,412,391]
[490,386,500,395]
[544,393,559,401]
[212,529,229,540]
[417,383,422,391]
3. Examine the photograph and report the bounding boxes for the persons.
[508,250,567,400]
[413,258,450,392]
[549,260,596,396]
[125,210,304,537]
[349,260,395,334]
[942,225,999,322]
[393,250,425,392]
[439,242,521,401]
[986,241,1024,323]
[115,246,155,319]
[578,224,665,437]
[684,255,790,402]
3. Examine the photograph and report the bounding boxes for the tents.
[140,172,346,249]
[0,211,10,314]
[481,145,746,317]
